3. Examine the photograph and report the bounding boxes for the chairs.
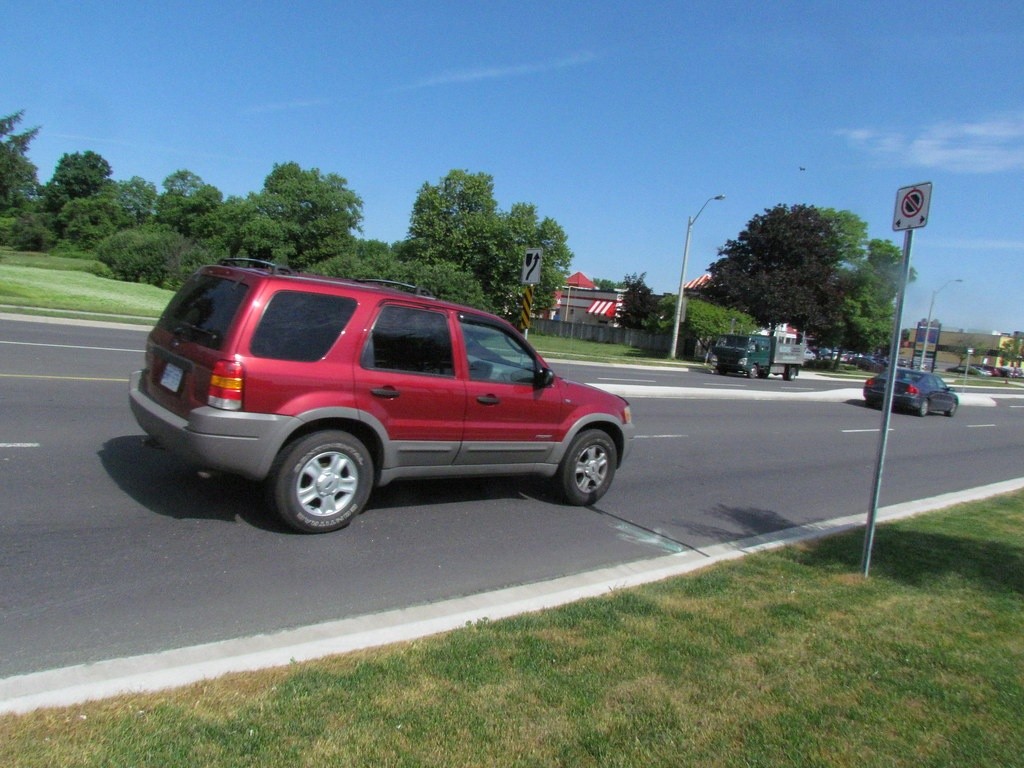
[912,376,919,381]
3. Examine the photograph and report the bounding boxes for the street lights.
[918,279,963,370]
[670,194,726,362]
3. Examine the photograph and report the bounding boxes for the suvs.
[126,256,639,535]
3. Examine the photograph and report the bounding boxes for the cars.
[803,345,909,372]
[945,363,1024,378]
[863,366,959,417]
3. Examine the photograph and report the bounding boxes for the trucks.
[707,332,806,382]
